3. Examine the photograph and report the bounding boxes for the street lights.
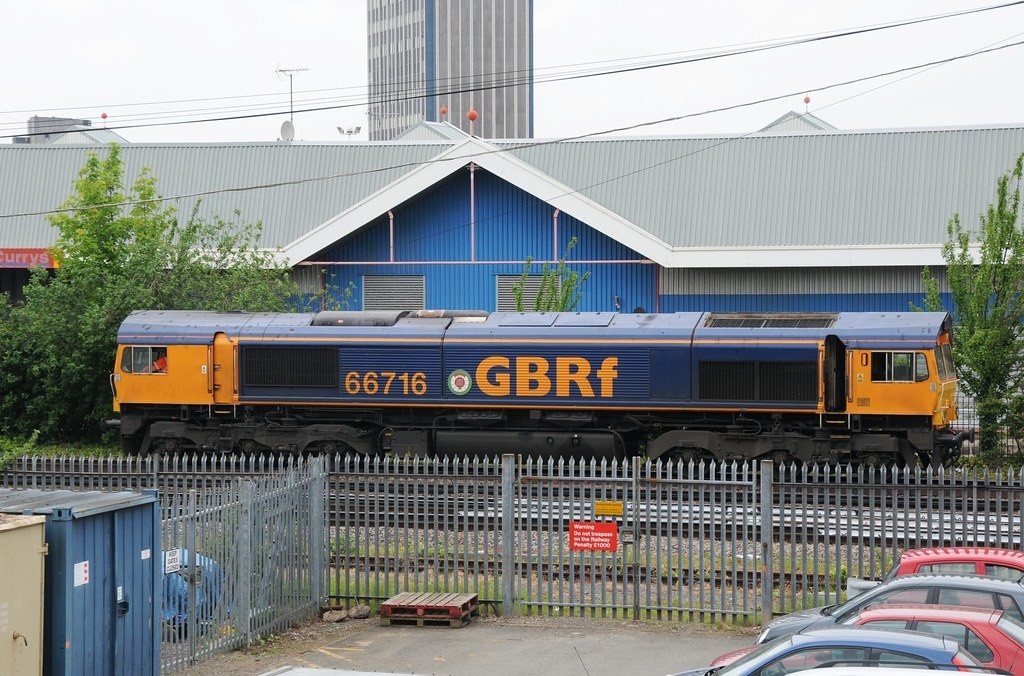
[337,124,365,142]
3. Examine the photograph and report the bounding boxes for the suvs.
[754,570,1024,664]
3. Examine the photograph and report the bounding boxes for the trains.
[108,309,974,490]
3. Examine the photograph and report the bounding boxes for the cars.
[660,625,996,676]
[883,547,1024,595]
[775,657,1013,676]
[708,605,1024,675]
